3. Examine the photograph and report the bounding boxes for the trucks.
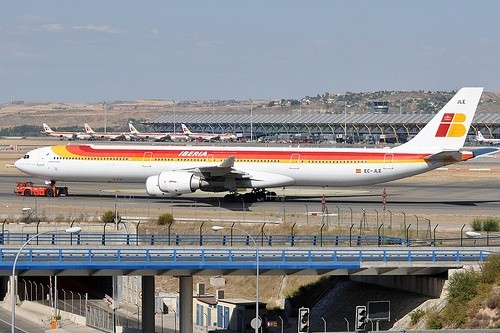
[14,181,58,197]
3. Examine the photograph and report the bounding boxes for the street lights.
[344,93,349,143]
[103,100,107,133]
[11,226,83,333]
[249,98,253,143]
[172,99,176,134]
[212,225,259,333]
[2,206,32,244]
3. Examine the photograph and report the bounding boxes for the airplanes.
[475,131,500,146]
[41,122,238,143]
[14,86,500,203]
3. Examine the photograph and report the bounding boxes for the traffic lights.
[355,306,367,331]
[298,308,310,333]
